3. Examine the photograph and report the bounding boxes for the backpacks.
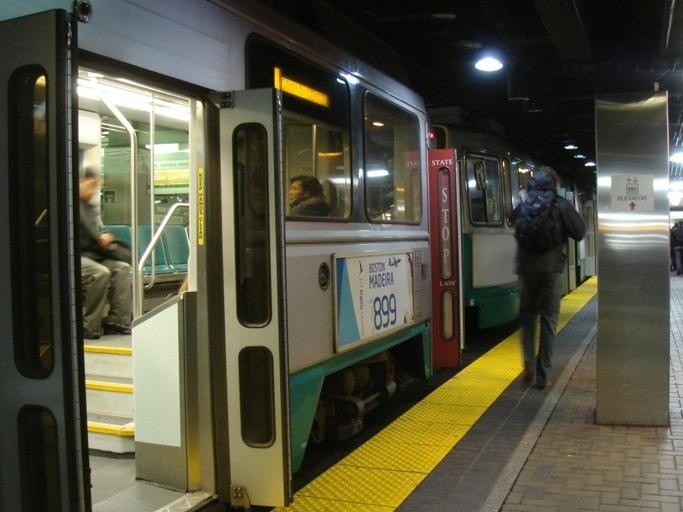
[514,189,567,254]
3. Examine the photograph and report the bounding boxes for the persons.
[508,166,586,389]
[670,221,682,276]
[78,168,132,339]
[287,175,329,215]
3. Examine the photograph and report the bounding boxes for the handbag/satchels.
[104,240,133,265]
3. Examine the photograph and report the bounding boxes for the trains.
[1,0,598,511]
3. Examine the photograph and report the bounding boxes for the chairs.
[98,223,188,288]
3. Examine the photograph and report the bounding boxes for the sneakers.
[523,369,552,388]
[83,321,132,339]
